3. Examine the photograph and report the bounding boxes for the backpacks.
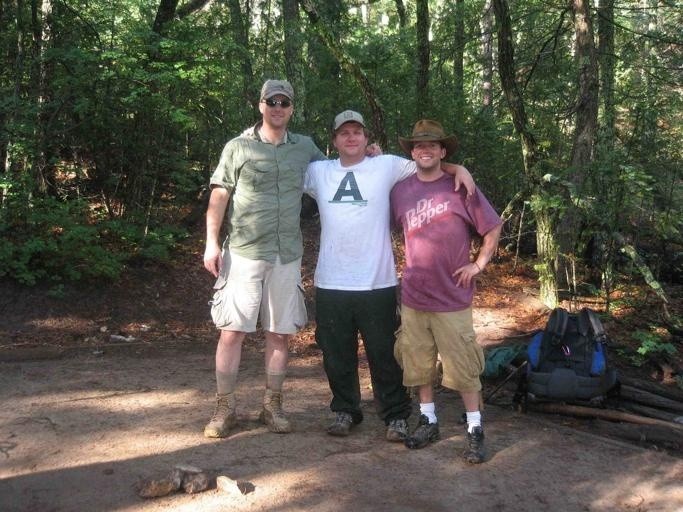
[518,306,620,408]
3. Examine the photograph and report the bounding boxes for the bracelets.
[475,262,482,272]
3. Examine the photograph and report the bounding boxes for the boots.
[260,389,292,433]
[204,392,236,438]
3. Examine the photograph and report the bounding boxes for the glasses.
[265,97,293,107]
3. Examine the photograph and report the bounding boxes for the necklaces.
[258,125,271,144]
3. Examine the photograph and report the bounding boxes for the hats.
[398,120,457,156]
[260,79,295,103]
[333,109,365,130]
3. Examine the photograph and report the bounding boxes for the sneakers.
[325,412,353,436]
[386,419,410,443]
[462,426,486,463]
[406,415,440,448]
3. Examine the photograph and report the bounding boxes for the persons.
[203,78,383,439]
[366,119,502,465]
[240,108,475,442]
